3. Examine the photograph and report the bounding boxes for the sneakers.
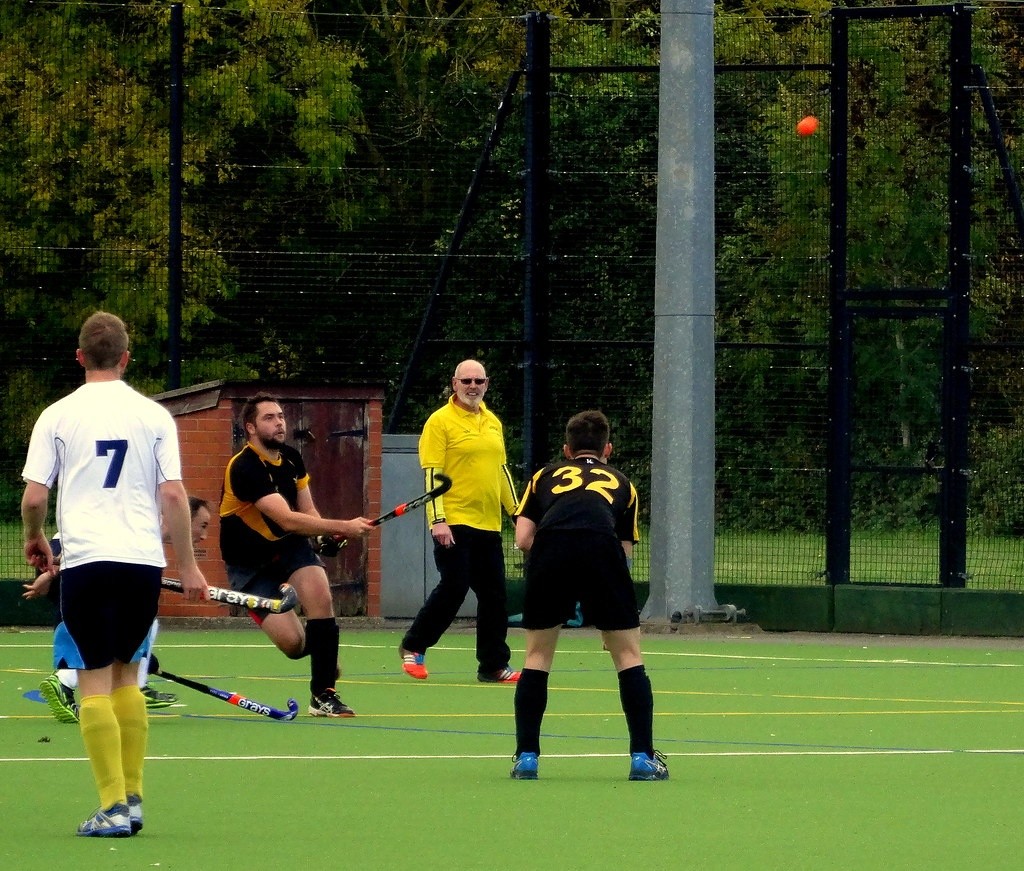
[510,752,538,779]
[127,793,144,834]
[398,641,428,678]
[78,802,131,838]
[139,685,180,708]
[39,670,80,723]
[628,748,669,780]
[308,687,355,717]
[476,664,521,683]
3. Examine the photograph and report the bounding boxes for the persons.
[217,392,376,717]
[398,359,522,683]
[22,497,211,723]
[510,410,670,781]
[21,311,211,837]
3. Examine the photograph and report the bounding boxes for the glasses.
[456,377,487,385]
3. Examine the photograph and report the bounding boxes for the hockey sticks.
[156,669,298,721]
[335,473,453,542]
[41,553,297,614]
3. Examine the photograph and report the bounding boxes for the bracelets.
[432,518,446,525]
